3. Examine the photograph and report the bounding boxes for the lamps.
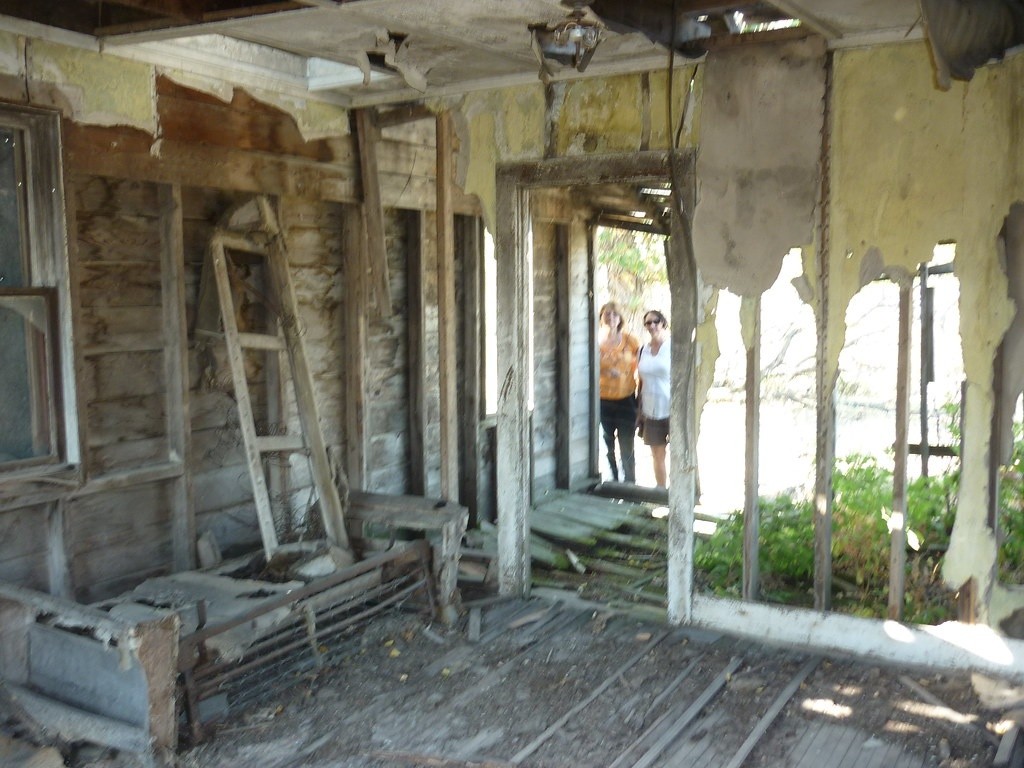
[527,0,608,84]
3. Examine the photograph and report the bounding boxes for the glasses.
[644,319,662,324]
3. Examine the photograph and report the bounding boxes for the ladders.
[202,189,351,560]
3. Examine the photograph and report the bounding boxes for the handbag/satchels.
[633,392,644,438]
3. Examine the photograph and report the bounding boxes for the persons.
[594,299,670,493]
